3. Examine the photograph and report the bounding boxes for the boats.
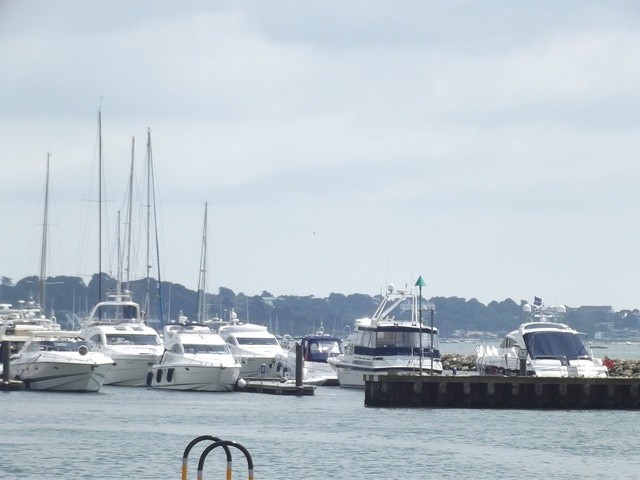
[327,283,444,388]
[287,321,343,386]
[475,296,608,378]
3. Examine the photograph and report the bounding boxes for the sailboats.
[0,153,114,391]
[197,200,285,377]
[140,126,242,392]
[79,112,165,387]
